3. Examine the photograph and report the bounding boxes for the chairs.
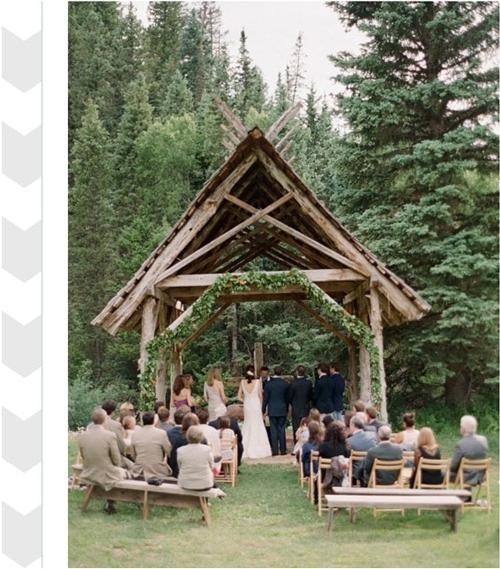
[412,457,452,516]
[456,456,493,516]
[316,455,355,516]
[368,456,406,519]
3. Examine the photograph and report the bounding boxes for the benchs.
[333,485,475,520]
[219,458,237,488]
[325,492,465,534]
[74,475,222,529]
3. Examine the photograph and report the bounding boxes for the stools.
[403,451,415,459]
[299,460,306,488]
[305,451,321,502]
[351,449,369,463]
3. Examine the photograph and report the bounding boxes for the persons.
[169,413,208,480]
[259,366,271,426]
[352,425,404,487]
[87,399,127,464]
[328,363,345,417]
[118,403,140,428]
[170,407,190,424]
[194,407,221,462]
[324,415,334,427]
[132,412,173,483]
[155,408,173,433]
[363,406,382,426]
[294,417,304,439]
[303,422,323,476]
[317,421,350,477]
[122,417,136,443]
[175,427,215,507]
[313,365,331,411]
[237,365,271,459]
[413,427,442,488]
[204,368,229,419]
[344,417,378,483]
[150,403,164,421]
[344,411,352,426]
[209,417,235,461]
[224,412,243,474]
[261,366,292,456]
[393,413,420,449]
[352,401,367,423]
[174,374,192,408]
[296,408,323,450]
[449,413,489,506]
[288,366,311,443]
[78,408,132,513]
